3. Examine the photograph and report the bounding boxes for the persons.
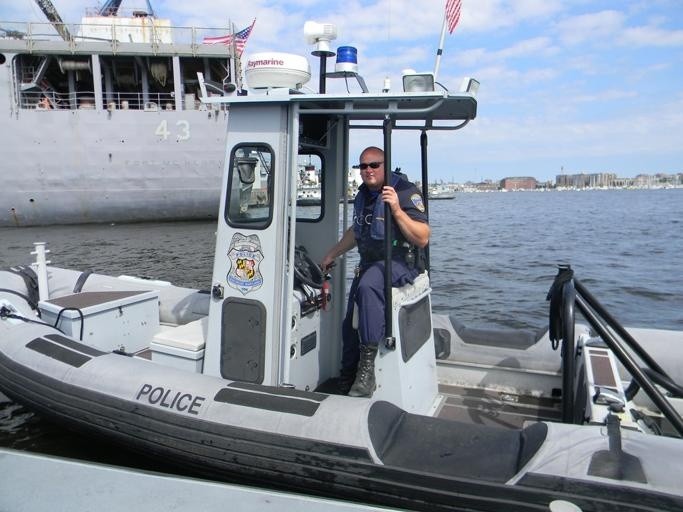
[318,147,430,398]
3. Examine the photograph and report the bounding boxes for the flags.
[203,22,256,59]
[445,0,464,34]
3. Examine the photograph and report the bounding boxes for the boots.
[335,344,378,398]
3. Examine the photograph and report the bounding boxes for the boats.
[0,21,683,508]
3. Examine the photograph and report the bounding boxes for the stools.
[151,314,209,373]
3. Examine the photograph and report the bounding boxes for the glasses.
[359,162,384,169]
